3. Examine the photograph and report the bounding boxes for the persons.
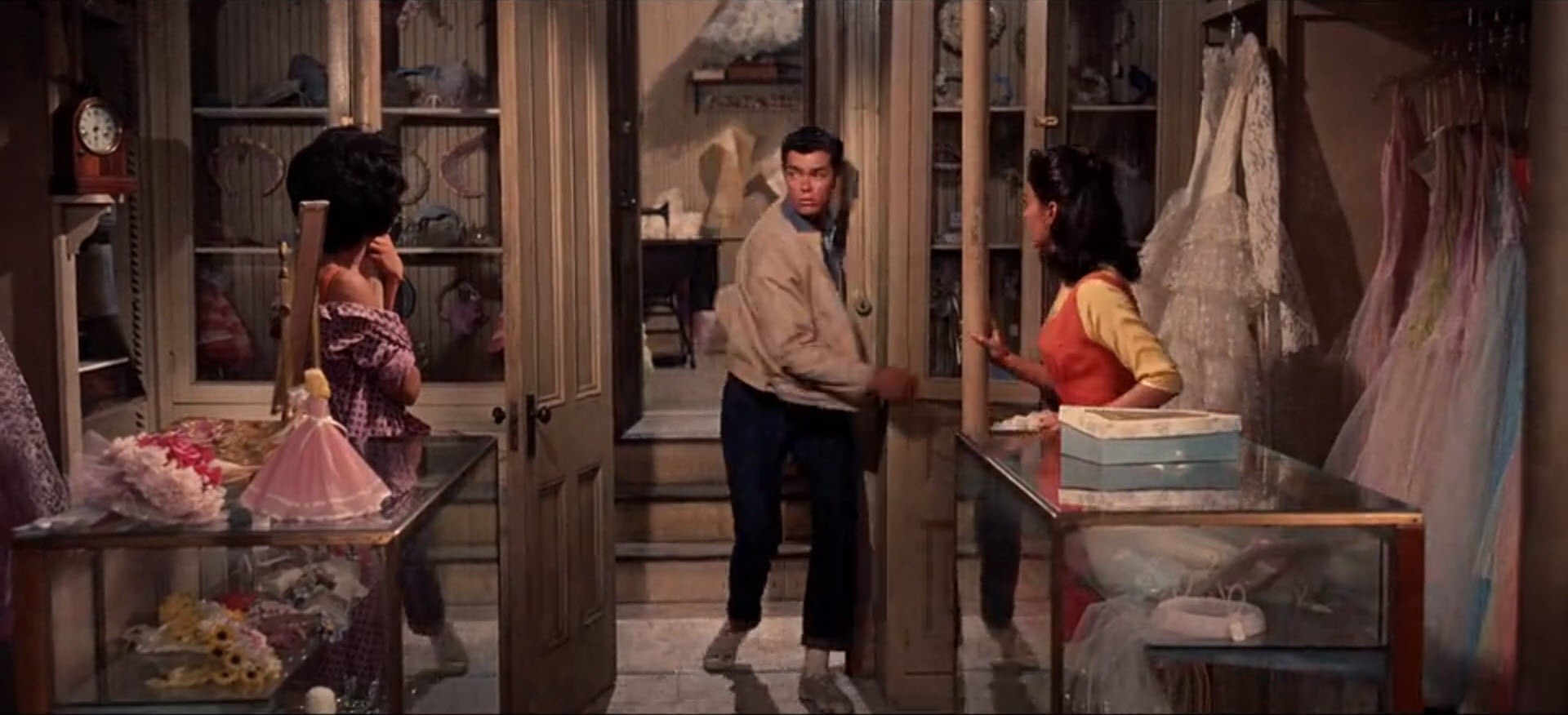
[974,141,1186,643]
[278,117,433,709]
[700,123,925,713]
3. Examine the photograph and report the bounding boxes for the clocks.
[51,92,140,198]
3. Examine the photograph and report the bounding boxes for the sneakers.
[798,667,855,715]
[430,621,469,676]
[986,624,1041,670]
[701,621,745,671]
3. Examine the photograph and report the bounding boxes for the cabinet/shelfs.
[157,11,498,682]
[921,1,1173,413]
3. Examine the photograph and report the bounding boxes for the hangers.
[1368,3,1534,114]
[1209,1,1248,58]
